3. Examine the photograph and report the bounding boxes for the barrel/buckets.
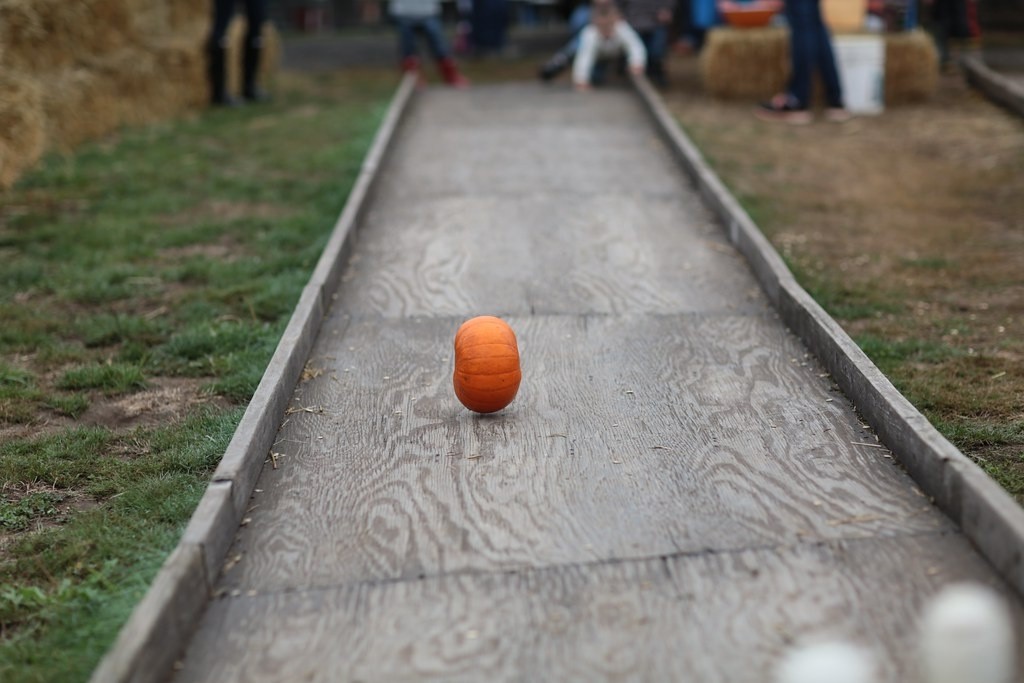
[831,40,885,116]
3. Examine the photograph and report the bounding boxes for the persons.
[386,0,471,90]
[202,0,272,110]
[538,0,719,96]
[764,0,850,124]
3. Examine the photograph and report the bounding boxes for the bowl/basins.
[724,10,779,28]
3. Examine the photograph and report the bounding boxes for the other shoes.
[442,59,469,88]
[243,86,264,107]
[212,90,230,106]
[762,93,809,116]
[402,55,419,68]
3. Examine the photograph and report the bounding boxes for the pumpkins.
[453,315,521,413]
